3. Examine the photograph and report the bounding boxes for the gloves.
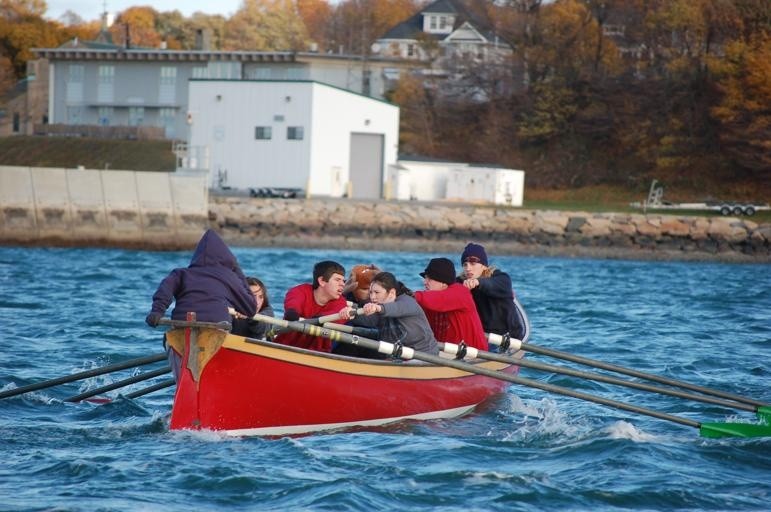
[146,313,162,327]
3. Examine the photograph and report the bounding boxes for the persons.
[456,243,523,337]
[272,260,348,354]
[339,272,440,359]
[232,276,274,340]
[145,230,258,331]
[407,258,489,357]
[342,265,411,308]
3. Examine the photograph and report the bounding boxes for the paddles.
[229,308,771,441]
[299,316,771,422]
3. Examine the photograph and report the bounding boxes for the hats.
[352,265,373,289]
[420,258,456,285]
[462,243,488,266]
